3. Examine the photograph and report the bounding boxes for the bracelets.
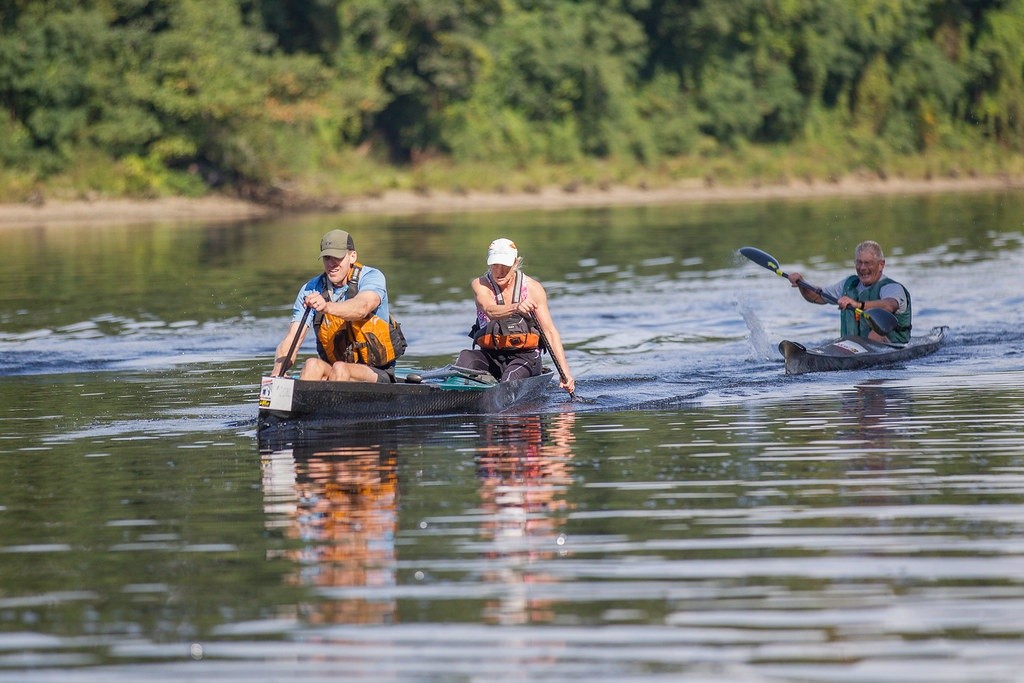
[859,301,866,309]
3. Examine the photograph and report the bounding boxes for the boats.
[256,366,554,427]
[778,324,948,374]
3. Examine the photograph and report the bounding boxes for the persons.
[787,240,912,345]
[454,238,575,395]
[269,228,408,384]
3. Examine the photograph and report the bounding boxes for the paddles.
[529,310,577,402]
[740,246,898,337]
[278,295,310,379]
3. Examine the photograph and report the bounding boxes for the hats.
[486,238,518,266]
[318,229,354,260]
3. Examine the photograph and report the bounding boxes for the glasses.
[854,258,882,266]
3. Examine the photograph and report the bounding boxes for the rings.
[316,303,319,306]
